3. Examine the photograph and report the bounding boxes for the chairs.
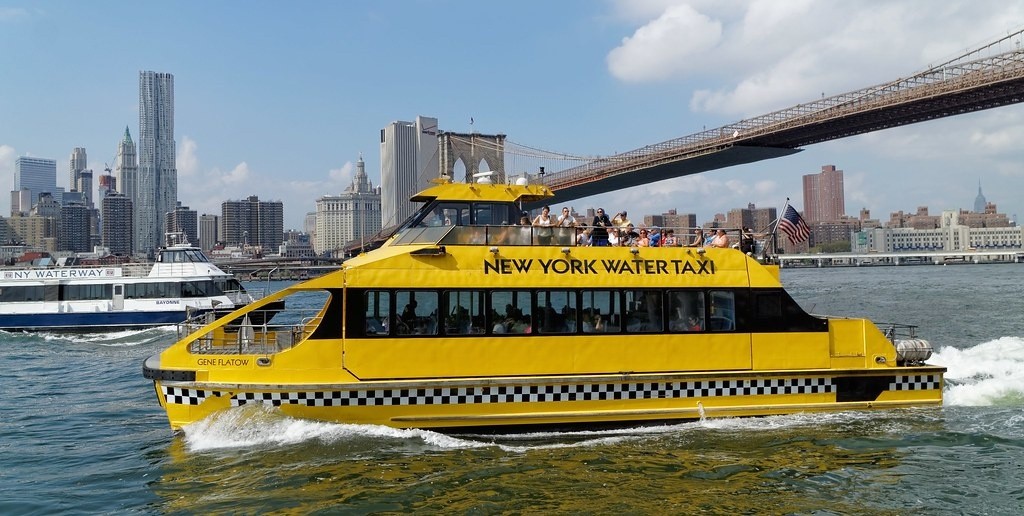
[627,318,643,331]
[699,319,705,330]
[562,319,576,332]
[583,321,593,332]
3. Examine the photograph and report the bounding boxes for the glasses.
[640,232,645,234]
[694,229,700,231]
[651,230,654,231]
[564,211,569,212]
[597,211,602,213]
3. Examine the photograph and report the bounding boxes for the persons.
[682,226,729,248]
[592,208,613,246]
[557,206,574,245]
[571,223,682,247]
[531,205,555,245]
[468,217,531,245]
[611,210,631,234]
[382,300,702,331]
[431,208,450,226]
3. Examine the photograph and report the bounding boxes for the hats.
[620,211,627,215]
[649,226,658,230]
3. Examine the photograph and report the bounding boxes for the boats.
[299,272,309,280]
[0,227,285,334]
[140,169,946,435]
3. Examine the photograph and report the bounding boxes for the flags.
[778,204,810,246]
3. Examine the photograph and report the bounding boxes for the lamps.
[561,248,572,257]
[630,249,640,258]
[696,248,708,257]
[490,248,500,255]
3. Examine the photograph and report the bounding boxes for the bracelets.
[688,244,690,246]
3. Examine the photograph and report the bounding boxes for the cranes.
[105,152,118,193]
